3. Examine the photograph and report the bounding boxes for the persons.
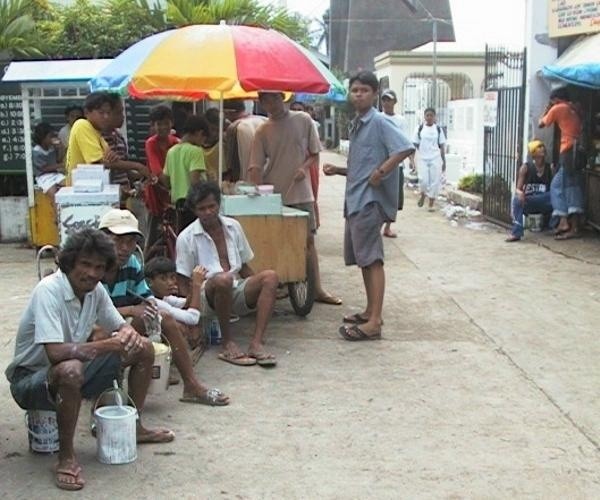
[408,107,451,213]
[323,69,418,343]
[536,87,587,241]
[32,92,343,308]
[377,89,416,239]
[96,208,233,408]
[503,138,559,243]
[172,179,280,368]
[3,227,178,492]
[145,255,211,386]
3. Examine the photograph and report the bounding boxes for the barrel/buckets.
[525,212,545,234]
[94,387,139,466]
[23,409,60,454]
[204,317,222,346]
[146,332,172,394]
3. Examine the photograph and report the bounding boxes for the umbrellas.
[86,19,347,194]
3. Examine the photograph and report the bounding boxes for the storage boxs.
[53,183,122,250]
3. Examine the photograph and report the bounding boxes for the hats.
[97,208,144,240]
[381,90,396,100]
[528,140,544,159]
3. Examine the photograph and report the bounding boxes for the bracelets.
[377,167,386,176]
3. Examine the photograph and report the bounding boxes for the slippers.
[248,352,276,365]
[383,233,396,238]
[418,199,435,212]
[504,227,582,243]
[52,462,85,491]
[344,313,383,326]
[339,326,382,340]
[218,351,257,365]
[137,426,175,442]
[315,295,343,304]
[179,388,231,406]
